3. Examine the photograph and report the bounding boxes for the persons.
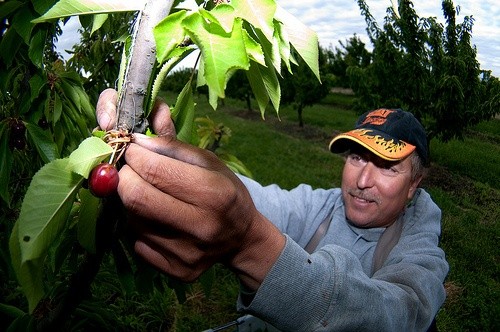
[93,85,450,332]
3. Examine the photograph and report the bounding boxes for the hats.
[327,108,429,167]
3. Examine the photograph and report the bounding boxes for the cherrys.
[10,114,50,146]
[88,163,118,196]
